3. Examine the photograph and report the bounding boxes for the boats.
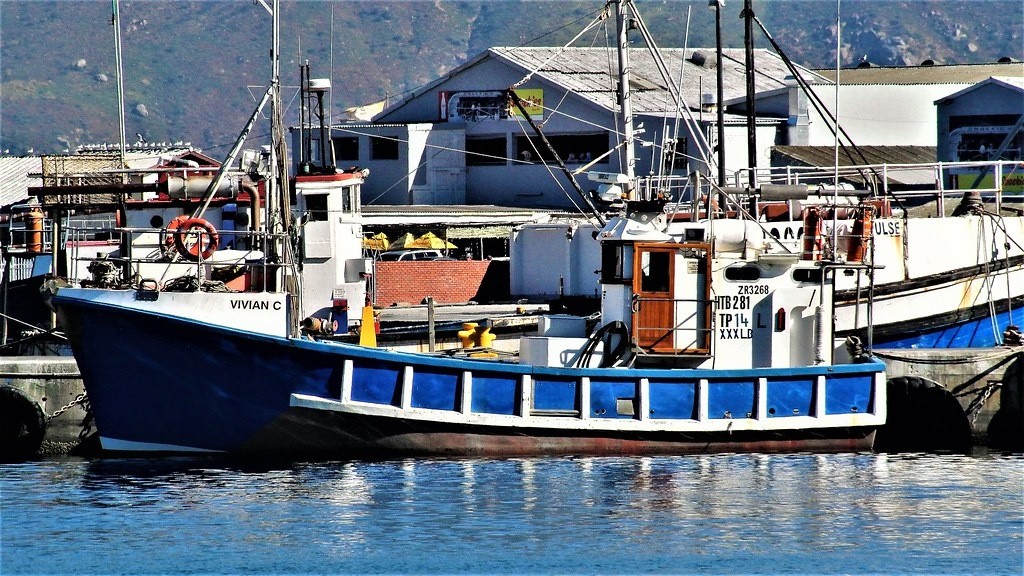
[0,1,1023,479]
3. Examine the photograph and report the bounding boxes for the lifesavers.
[166,214,205,257]
[176,218,219,261]
